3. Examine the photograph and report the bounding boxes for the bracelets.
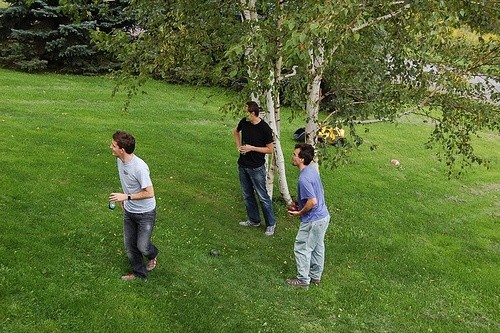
[128,193,131,200]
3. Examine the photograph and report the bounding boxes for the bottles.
[109,190,117,210]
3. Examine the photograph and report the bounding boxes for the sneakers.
[286,278,310,288]
[239,220,260,227]
[146,256,157,271]
[265,223,276,235]
[121,273,135,279]
[311,279,320,283]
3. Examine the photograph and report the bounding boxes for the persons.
[109,130,159,279]
[293,127,305,139]
[286,143,330,289]
[234,101,276,235]
